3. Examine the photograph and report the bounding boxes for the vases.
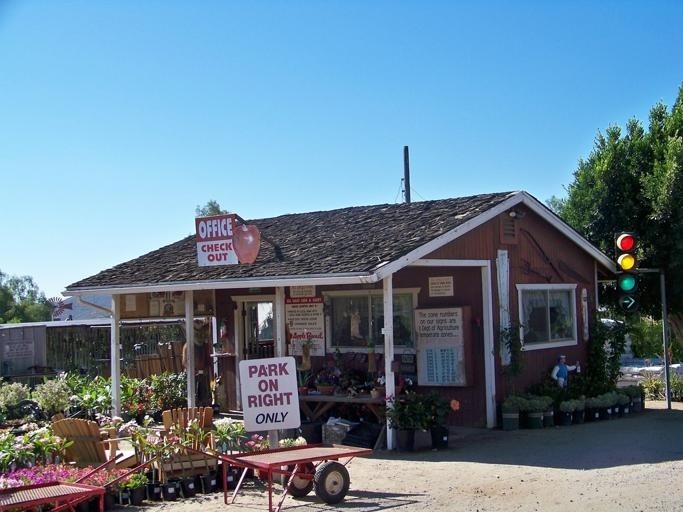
[254,465,271,481]
[104,415,246,509]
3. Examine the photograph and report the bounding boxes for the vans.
[598,317,636,363]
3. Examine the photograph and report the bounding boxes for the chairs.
[49,418,143,475]
[146,403,218,504]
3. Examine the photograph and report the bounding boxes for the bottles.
[576,361,580,373]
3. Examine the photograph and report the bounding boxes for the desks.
[294,391,411,452]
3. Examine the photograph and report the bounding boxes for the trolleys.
[168,438,374,512]
[0,452,158,511]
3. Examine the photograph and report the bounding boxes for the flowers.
[244,433,266,454]
[0,464,104,511]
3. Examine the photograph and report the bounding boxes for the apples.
[233,224,260,264]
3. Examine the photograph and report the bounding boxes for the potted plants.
[383,391,465,451]
[296,366,405,394]
[60,370,187,427]
[499,384,646,432]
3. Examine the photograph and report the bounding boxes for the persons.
[179,328,212,405]
[549,353,581,387]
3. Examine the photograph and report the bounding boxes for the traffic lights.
[615,229,639,313]
